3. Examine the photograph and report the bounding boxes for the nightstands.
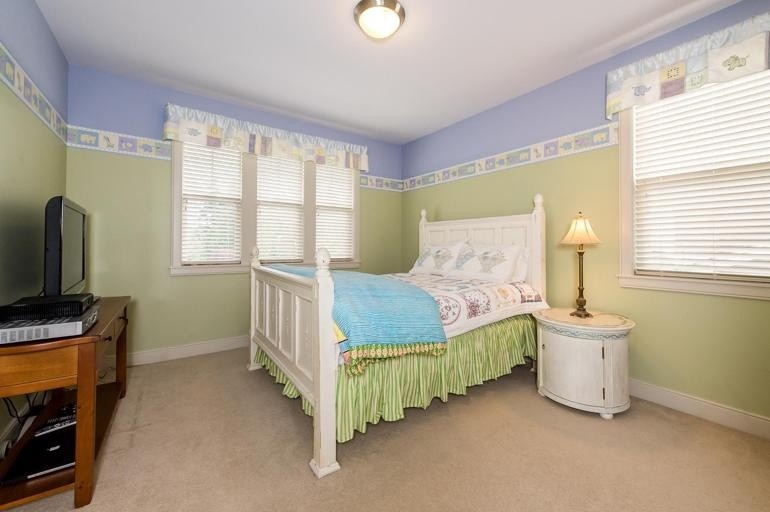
[533,307,635,420]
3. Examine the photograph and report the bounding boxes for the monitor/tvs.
[44,196,87,295]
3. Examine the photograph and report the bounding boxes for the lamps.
[557,210,603,319]
[352,0,406,42]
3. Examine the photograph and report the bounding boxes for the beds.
[245,193,550,478]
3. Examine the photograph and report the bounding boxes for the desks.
[0,297,129,509]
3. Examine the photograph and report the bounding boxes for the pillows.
[446,238,520,283]
[409,241,460,279]
[513,245,532,284]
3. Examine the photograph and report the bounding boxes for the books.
[34,400,76,437]
